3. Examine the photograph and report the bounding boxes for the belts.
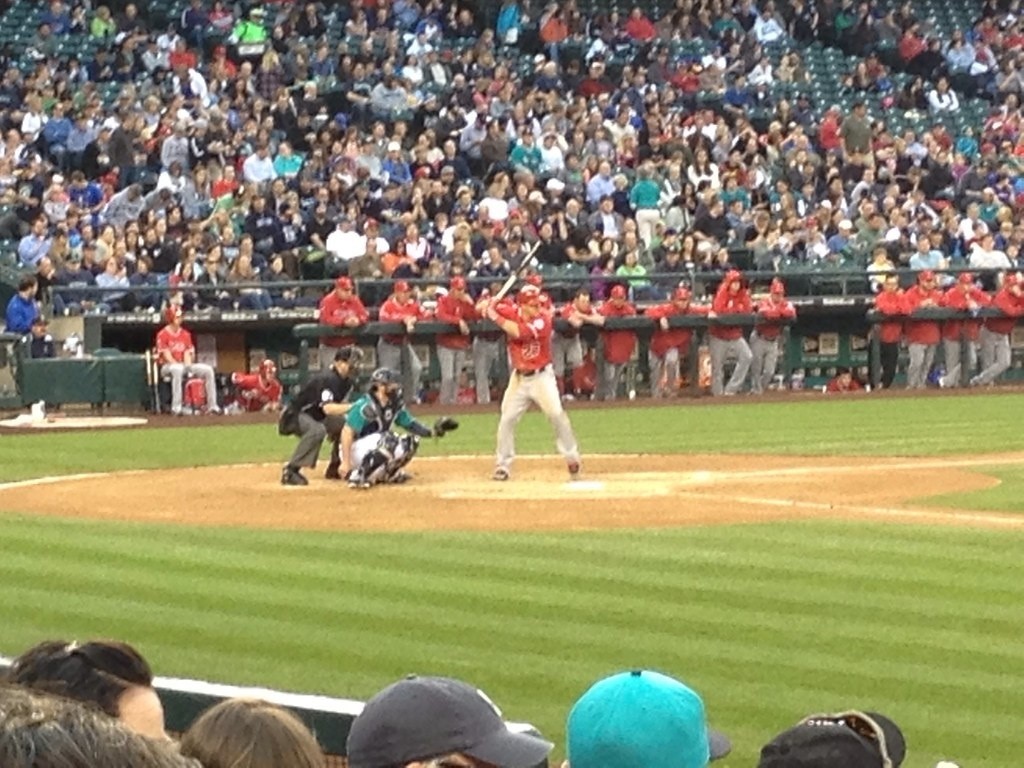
[516,367,546,377]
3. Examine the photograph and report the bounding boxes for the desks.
[101,352,159,404]
[18,354,103,409]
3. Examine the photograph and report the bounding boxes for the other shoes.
[281,468,308,484]
[327,465,340,479]
[376,473,408,485]
[568,461,583,477]
[349,474,371,488]
[494,470,508,480]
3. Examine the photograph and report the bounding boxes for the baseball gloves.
[432,416,458,437]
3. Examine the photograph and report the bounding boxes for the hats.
[337,277,354,290]
[566,669,731,768]
[395,281,410,293]
[516,285,548,306]
[757,710,905,768]
[337,346,363,361]
[167,306,185,317]
[449,277,466,289]
[610,270,1021,302]
[369,368,401,386]
[345,674,555,768]
[261,360,277,372]
[32,316,50,325]
[379,129,1013,250]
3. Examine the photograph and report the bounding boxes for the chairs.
[3,0,1024,415]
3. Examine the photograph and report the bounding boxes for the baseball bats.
[144,348,162,415]
[494,240,543,303]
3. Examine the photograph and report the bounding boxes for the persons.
[0,0,1024,424]
[0,636,961,768]
[277,341,364,486]
[337,367,459,491]
[475,284,584,482]
